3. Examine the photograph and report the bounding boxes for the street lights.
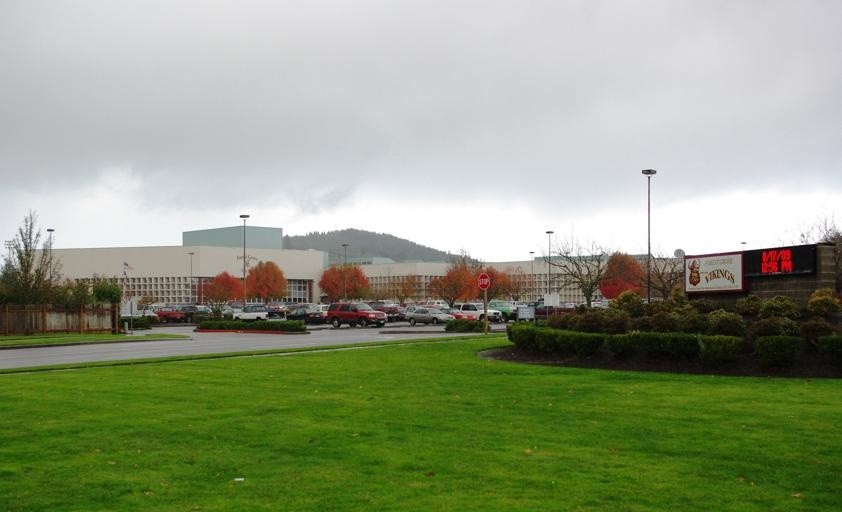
[238,213,250,310]
[186,251,196,305]
[46,227,54,283]
[340,242,350,301]
[544,231,554,297]
[528,251,535,300]
[641,168,658,305]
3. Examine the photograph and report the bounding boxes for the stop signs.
[475,272,492,293]
